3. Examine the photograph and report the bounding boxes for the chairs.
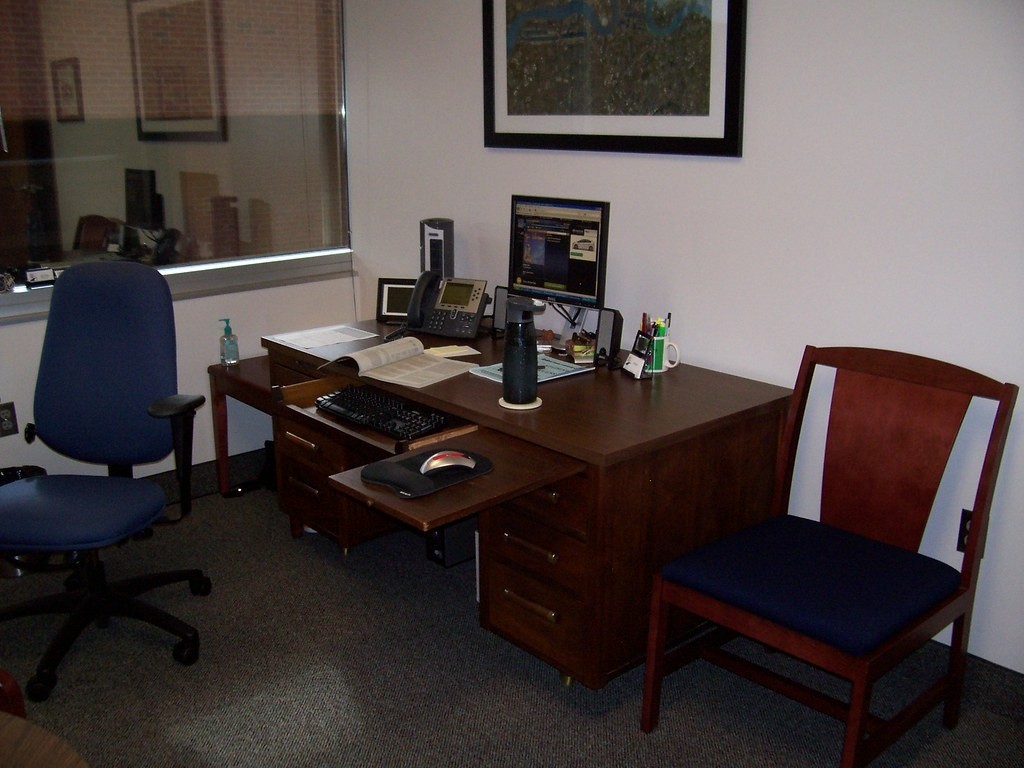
[0,260,201,703]
[641,345,1019,768]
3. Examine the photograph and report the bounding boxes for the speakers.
[491,286,509,340]
[594,307,623,370]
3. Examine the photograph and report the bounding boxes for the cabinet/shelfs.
[479,413,778,689]
[269,349,406,558]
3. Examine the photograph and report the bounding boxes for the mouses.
[419,450,476,477]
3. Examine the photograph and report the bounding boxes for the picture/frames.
[377,278,416,323]
[51,58,85,124]
[121,0,229,145]
[480,0,748,160]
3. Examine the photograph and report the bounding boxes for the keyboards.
[315,385,452,442]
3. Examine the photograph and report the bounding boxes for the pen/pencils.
[639,311,672,336]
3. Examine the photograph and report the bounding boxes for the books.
[468,352,595,386]
[316,336,474,389]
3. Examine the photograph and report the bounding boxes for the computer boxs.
[426,515,481,569]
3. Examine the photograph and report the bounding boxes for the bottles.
[502,297,546,404]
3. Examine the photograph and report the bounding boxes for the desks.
[206,324,796,690]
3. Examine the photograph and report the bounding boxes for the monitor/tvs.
[508,194,610,352]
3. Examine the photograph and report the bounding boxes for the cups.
[643,336,679,373]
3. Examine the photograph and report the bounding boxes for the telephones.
[403,272,492,340]
